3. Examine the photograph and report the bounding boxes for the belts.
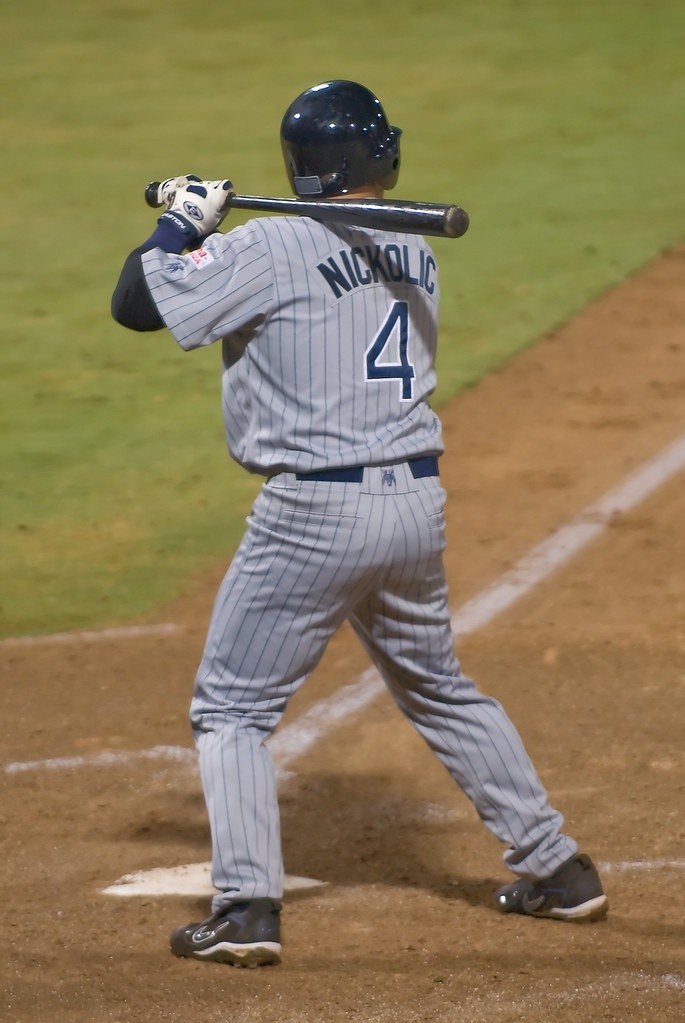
[296,457,439,482]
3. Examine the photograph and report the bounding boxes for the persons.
[110,82,609,971]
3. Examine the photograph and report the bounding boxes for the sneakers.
[494,854,610,922]
[169,898,282,968]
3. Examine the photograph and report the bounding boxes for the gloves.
[157,173,236,252]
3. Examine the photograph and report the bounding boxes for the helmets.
[279,80,401,198]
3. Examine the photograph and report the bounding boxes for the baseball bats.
[140,174,476,241]
